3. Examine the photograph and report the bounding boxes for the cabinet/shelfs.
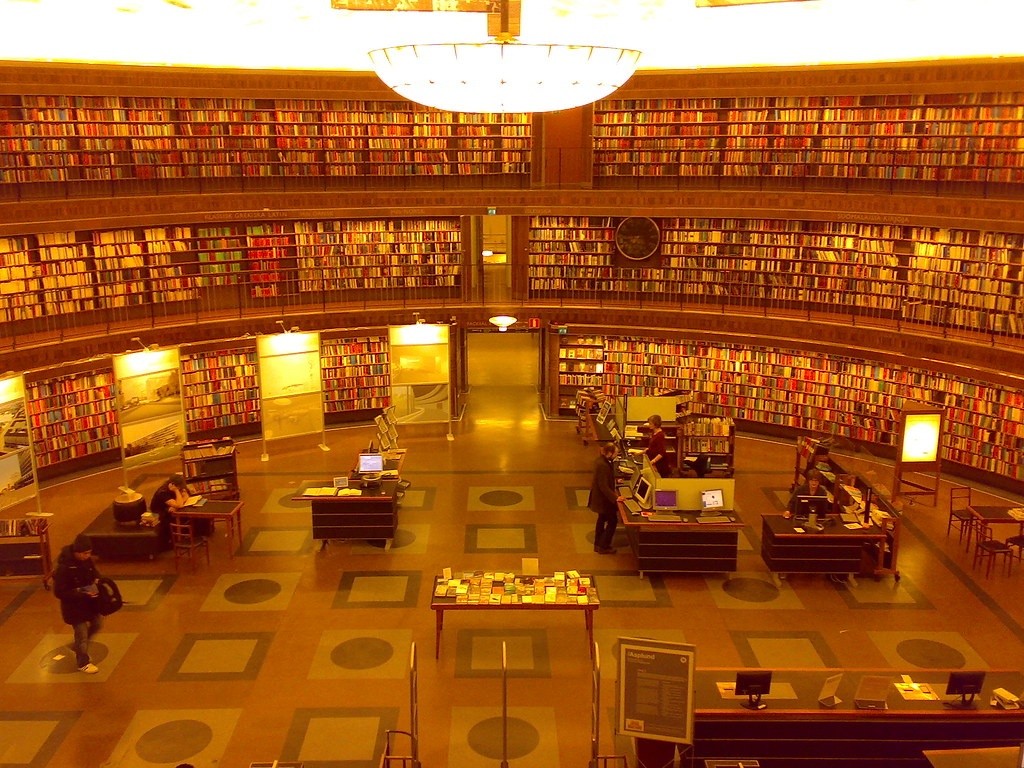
[0,518,55,590]
[0,55,1024,500]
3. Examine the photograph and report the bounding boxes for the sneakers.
[70,649,76,656]
[77,663,99,674]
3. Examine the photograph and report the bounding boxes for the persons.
[54,533,103,674]
[586,442,627,554]
[634,415,669,478]
[781,468,828,521]
[150,474,189,542]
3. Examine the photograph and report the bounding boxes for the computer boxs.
[360,479,383,489]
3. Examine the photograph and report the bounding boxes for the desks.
[293,479,400,551]
[761,513,887,584]
[171,499,245,560]
[430,574,600,660]
[611,478,745,580]
[965,504,1024,555]
[633,670,1024,768]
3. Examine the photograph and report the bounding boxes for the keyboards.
[648,515,681,523]
[623,499,642,512]
[696,515,731,523]
[619,487,633,499]
[632,453,644,465]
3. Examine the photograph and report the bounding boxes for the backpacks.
[96,577,123,616]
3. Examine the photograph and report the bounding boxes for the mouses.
[631,512,640,516]
[784,515,790,518]
[729,517,736,521]
[683,517,688,522]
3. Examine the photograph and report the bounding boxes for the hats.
[170,474,187,489]
[72,533,92,552]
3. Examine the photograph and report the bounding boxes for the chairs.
[948,486,976,541]
[972,521,1013,580]
[169,523,210,570]
[1004,527,1024,564]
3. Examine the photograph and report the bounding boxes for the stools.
[75,504,165,559]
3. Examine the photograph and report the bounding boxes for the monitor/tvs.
[735,670,772,710]
[613,432,678,515]
[358,454,384,481]
[795,494,828,530]
[367,440,379,453]
[946,671,986,709]
[700,489,724,517]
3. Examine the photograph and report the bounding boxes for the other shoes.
[594,545,617,554]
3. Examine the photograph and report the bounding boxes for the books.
[0,95,532,184]
[179,345,261,434]
[302,487,362,497]
[0,518,38,537]
[320,336,391,414]
[26,367,122,468]
[559,334,1024,481]
[375,407,407,476]
[526,212,1024,335]
[0,218,462,325]
[1007,507,1024,521]
[593,90,1024,184]
[840,484,894,530]
[183,445,235,507]
[434,567,601,605]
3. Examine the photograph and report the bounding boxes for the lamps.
[367,0,642,115]
